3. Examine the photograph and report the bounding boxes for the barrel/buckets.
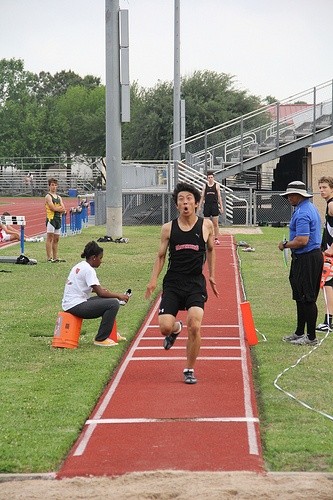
[52,312,83,349]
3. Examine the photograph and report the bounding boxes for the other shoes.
[47,258,66,263]
[315,323,333,333]
[289,337,319,345]
[214,239,220,245]
[283,333,306,342]
[32,237,44,243]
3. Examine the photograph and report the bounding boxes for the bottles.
[119,289,131,306]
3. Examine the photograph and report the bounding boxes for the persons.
[315,177,333,332]
[279,181,324,345]
[199,173,222,245]
[0,212,43,242]
[45,178,65,263]
[145,182,219,385]
[62,241,129,346]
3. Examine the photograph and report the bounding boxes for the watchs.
[282,241,287,248]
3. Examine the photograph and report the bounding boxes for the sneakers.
[93,337,120,346]
[163,320,184,351]
[182,368,197,384]
[117,332,127,342]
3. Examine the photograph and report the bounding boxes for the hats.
[279,181,314,198]
